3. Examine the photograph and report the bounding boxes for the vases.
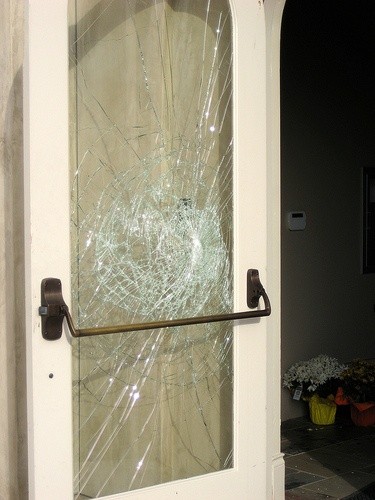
[308,394,337,425]
[350,400,374,427]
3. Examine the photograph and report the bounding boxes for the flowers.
[282,352,352,401]
[338,357,375,404]
